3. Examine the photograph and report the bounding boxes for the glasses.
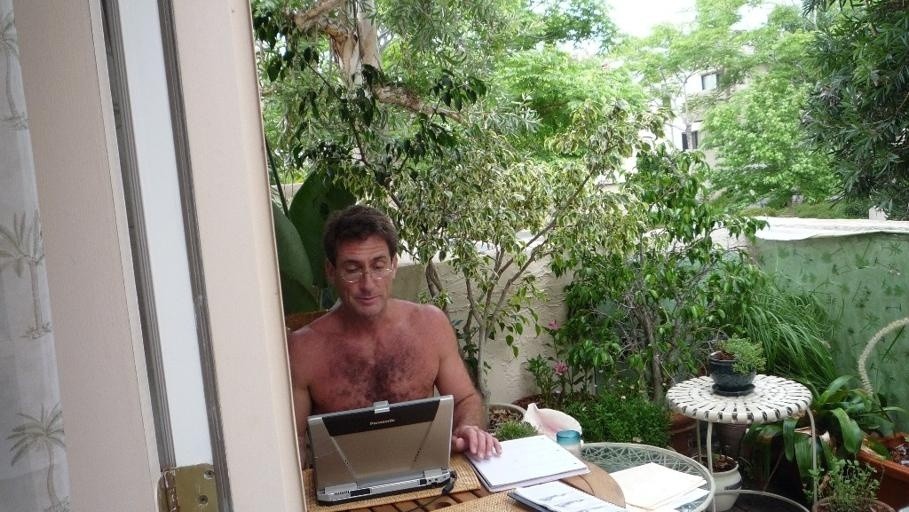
[333,257,396,284]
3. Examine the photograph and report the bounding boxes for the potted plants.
[245,0,909,511]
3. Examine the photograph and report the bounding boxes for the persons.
[286,204,503,474]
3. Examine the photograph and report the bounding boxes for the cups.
[556,430,582,459]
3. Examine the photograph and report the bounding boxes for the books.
[507,482,628,512]
[464,433,590,494]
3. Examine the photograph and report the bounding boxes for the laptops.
[306,395,455,505]
